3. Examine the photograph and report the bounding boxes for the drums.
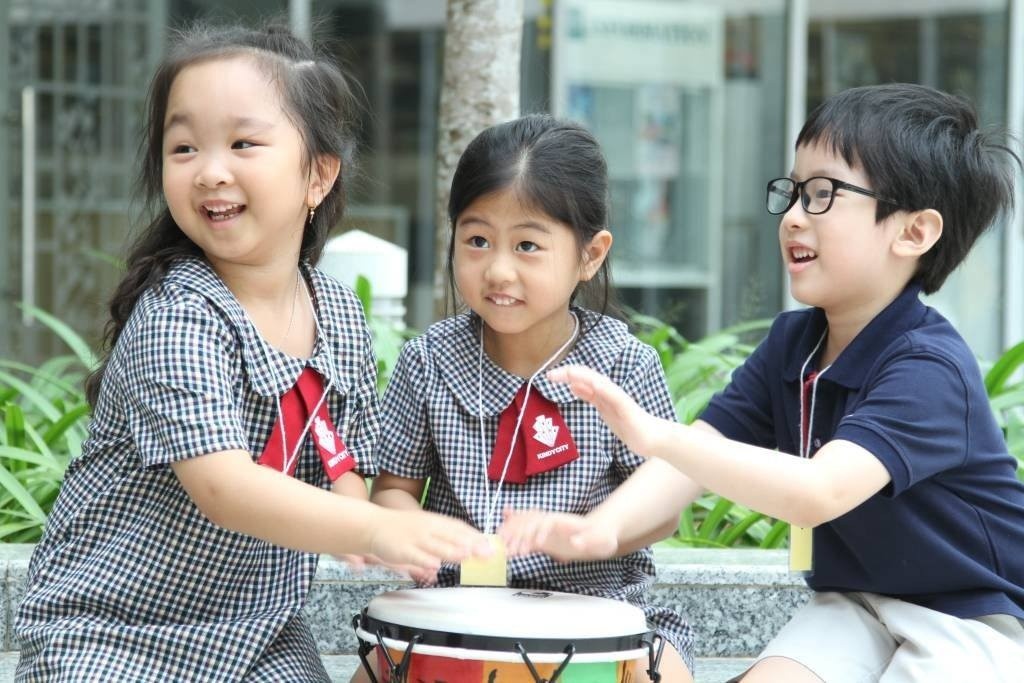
[352,586,665,683]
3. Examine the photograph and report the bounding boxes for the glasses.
[766,176,900,215]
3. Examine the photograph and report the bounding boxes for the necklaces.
[800,323,833,458]
[218,267,335,473]
[480,310,580,534]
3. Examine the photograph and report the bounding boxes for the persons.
[349,114,694,683]
[498,82,1024,683]
[15,24,492,683]
